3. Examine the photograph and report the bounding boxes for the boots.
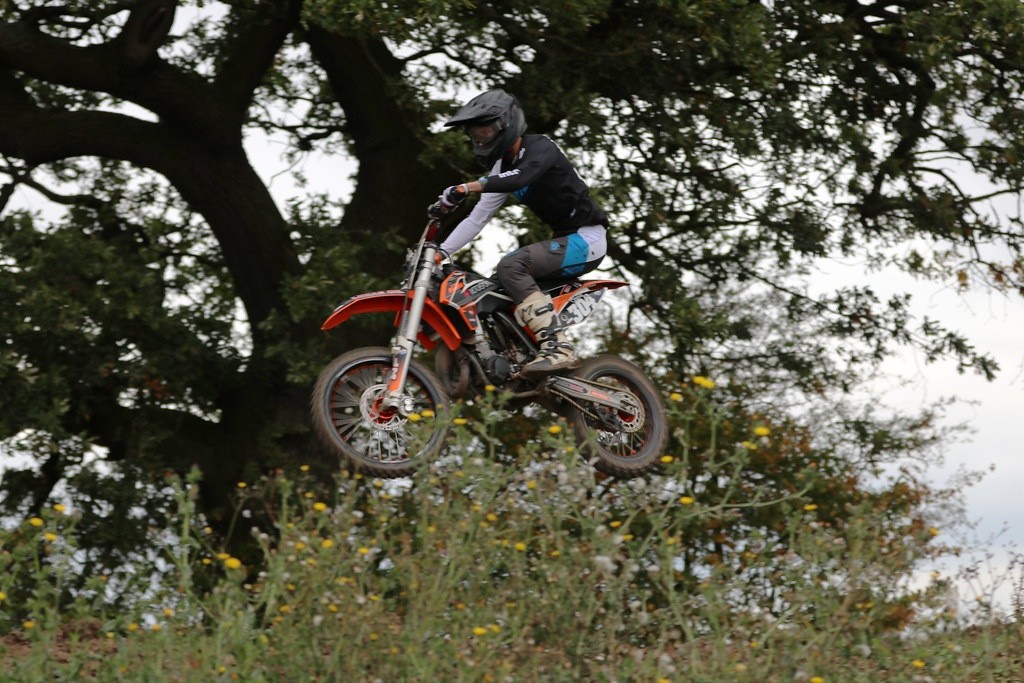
[517,294,584,375]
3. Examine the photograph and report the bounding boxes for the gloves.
[433,253,443,265]
[442,183,469,210]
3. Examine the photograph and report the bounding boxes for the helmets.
[444,89,528,168]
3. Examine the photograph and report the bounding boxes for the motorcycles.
[310,184,671,481]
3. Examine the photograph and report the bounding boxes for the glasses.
[469,121,502,147]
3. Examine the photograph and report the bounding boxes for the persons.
[429,89,611,374]
